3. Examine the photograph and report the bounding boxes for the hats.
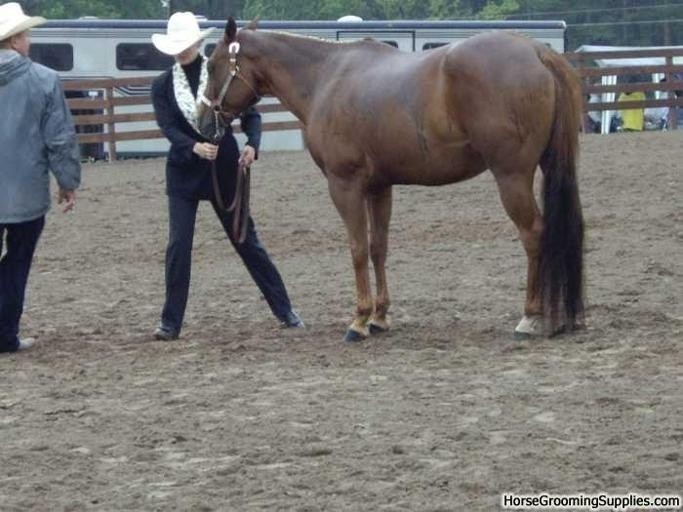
[151,11,216,54]
[0,3,47,41]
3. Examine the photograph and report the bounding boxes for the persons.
[0,2,82,354]
[577,76,683,134]
[150,11,306,341]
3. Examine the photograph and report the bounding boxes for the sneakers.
[152,328,177,340]
[284,311,304,327]
[17,336,33,351]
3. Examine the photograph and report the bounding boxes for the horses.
[196,16,587,344]
